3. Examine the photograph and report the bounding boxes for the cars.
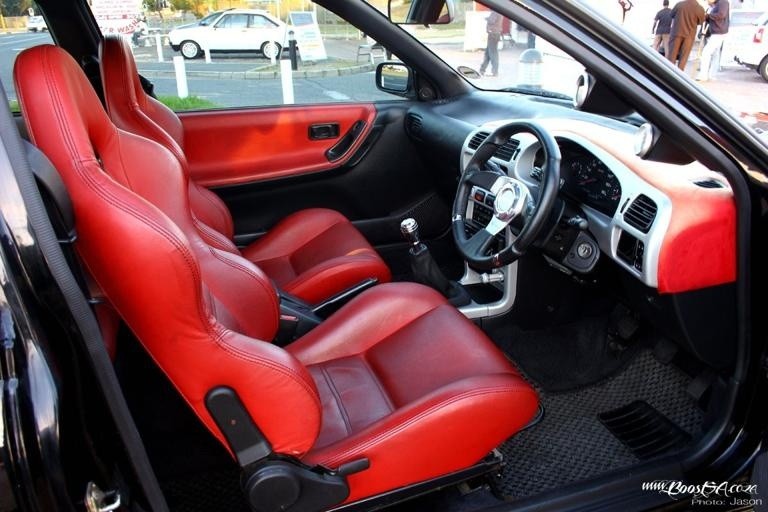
[166,9,297,60]
[0,0,768,511]
[170,8,233,29]
[732,11,768,82]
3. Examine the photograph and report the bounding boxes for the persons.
[696,0,729,81]
[131,16,148,48]
[668,0,705,71]
[479,11,503,77]
[652,0,675,58]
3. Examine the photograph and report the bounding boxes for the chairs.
[87,33,397,349]
[9,40,547,510]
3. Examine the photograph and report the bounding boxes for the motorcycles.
[129,25,153,48]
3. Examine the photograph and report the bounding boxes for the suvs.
[27,16,50,32]
